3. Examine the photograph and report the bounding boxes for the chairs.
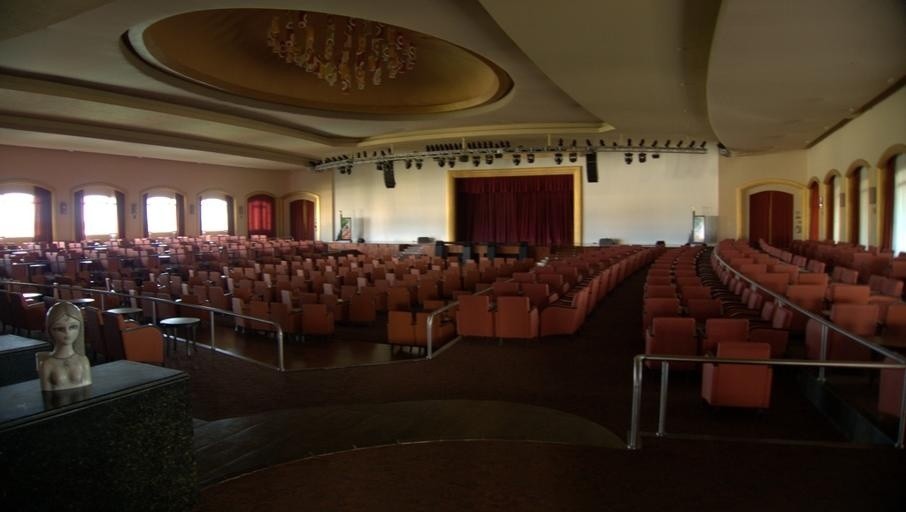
[199,261,308,300]
[232,272,347,336]
[455,245,666,346]
[338,253,443,324]
[711,237,906,420]
[442,255,534,292]
[0,290,46,335]
[142,286,231,318]
[386,299,446,357]
[60,269,185,298]
[85,306,104,363]
[642,246,776,409]
[103,312,164,368]
[0,234,326,283]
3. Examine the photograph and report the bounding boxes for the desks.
[0,359,199,511]
[0,334,51,385]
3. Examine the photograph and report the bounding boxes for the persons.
[40,301,92,389]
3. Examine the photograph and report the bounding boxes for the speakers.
[487,244,496,257]
[418,237,430,243]
[520,242,528,259]
[464,245,472,259]
[436,241,445,256]
[586,152,598,182]
[383,161,395,188]
[599,239,614,244]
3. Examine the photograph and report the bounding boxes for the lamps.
[266,11,417,92]
